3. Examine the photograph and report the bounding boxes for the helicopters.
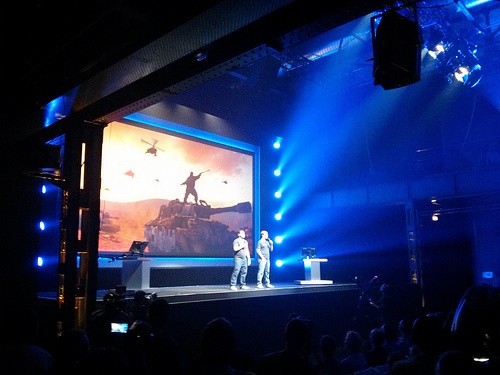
[140,136,165,156]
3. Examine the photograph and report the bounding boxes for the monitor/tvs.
[129,241,149,256]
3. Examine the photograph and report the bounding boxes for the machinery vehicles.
[143,198,251,257]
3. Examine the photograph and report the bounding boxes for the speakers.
[372,12,423,90]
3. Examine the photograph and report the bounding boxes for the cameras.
[109,321,129,334]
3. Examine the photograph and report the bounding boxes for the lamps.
[422,21,493,92]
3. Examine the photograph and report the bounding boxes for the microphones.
[268,238,270,241]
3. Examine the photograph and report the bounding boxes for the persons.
[179,171,204,205]
[1,282,500,375]
[230,230,251,291]
[255,230,274,289]
